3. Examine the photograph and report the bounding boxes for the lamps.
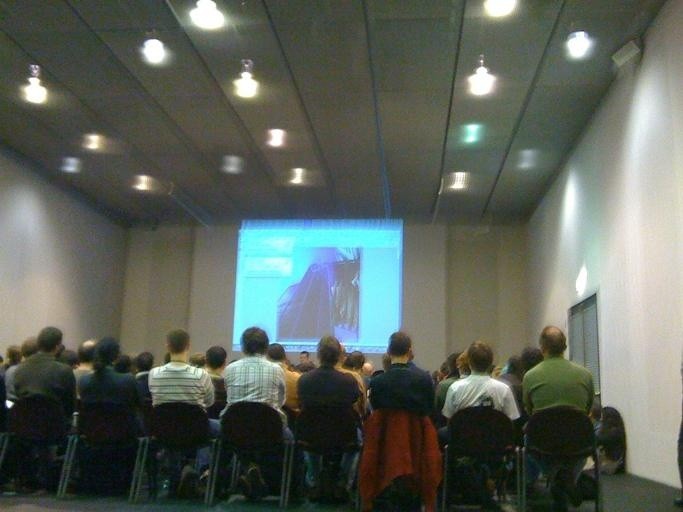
[468,53,497,95]
[564,30,588,58]
[142,30,164,64]
[233,59,259,99]
[189,0,225,31]
[22,65,46,103]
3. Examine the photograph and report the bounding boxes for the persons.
[673,364,683,507]
[1,327,627,511]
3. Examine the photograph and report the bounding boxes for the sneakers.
[240,474,251,495]
[248,463,262,499]
[177,465,196,494]
